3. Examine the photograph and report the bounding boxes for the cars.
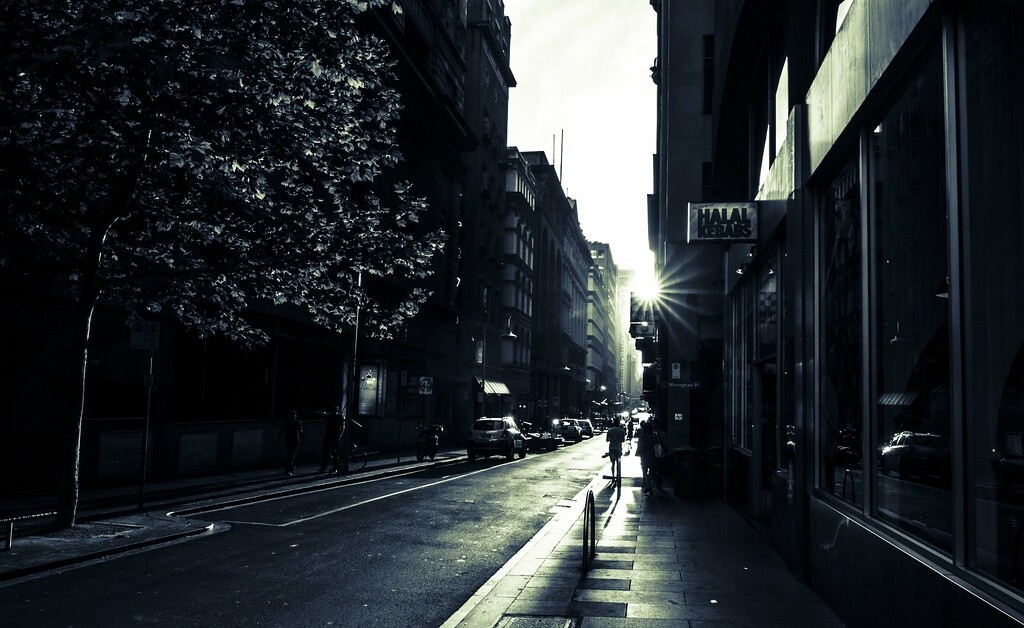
[837,445,864,469]
[523,425,557,450]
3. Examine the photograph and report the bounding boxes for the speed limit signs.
[419,376,433,394]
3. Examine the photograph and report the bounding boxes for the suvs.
[466,415,527,463]
[554,419,583,443]
[578,419,594,438]
[879,433,949,482]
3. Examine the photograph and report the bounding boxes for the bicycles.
[330,440,368,474]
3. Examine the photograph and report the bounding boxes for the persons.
[635,421,662,494]
[284,409,304,476]
[618,417,647,460]
[318,405,345,476]
[606,419,625,483]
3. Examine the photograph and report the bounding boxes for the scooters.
[415,420,445,461]
